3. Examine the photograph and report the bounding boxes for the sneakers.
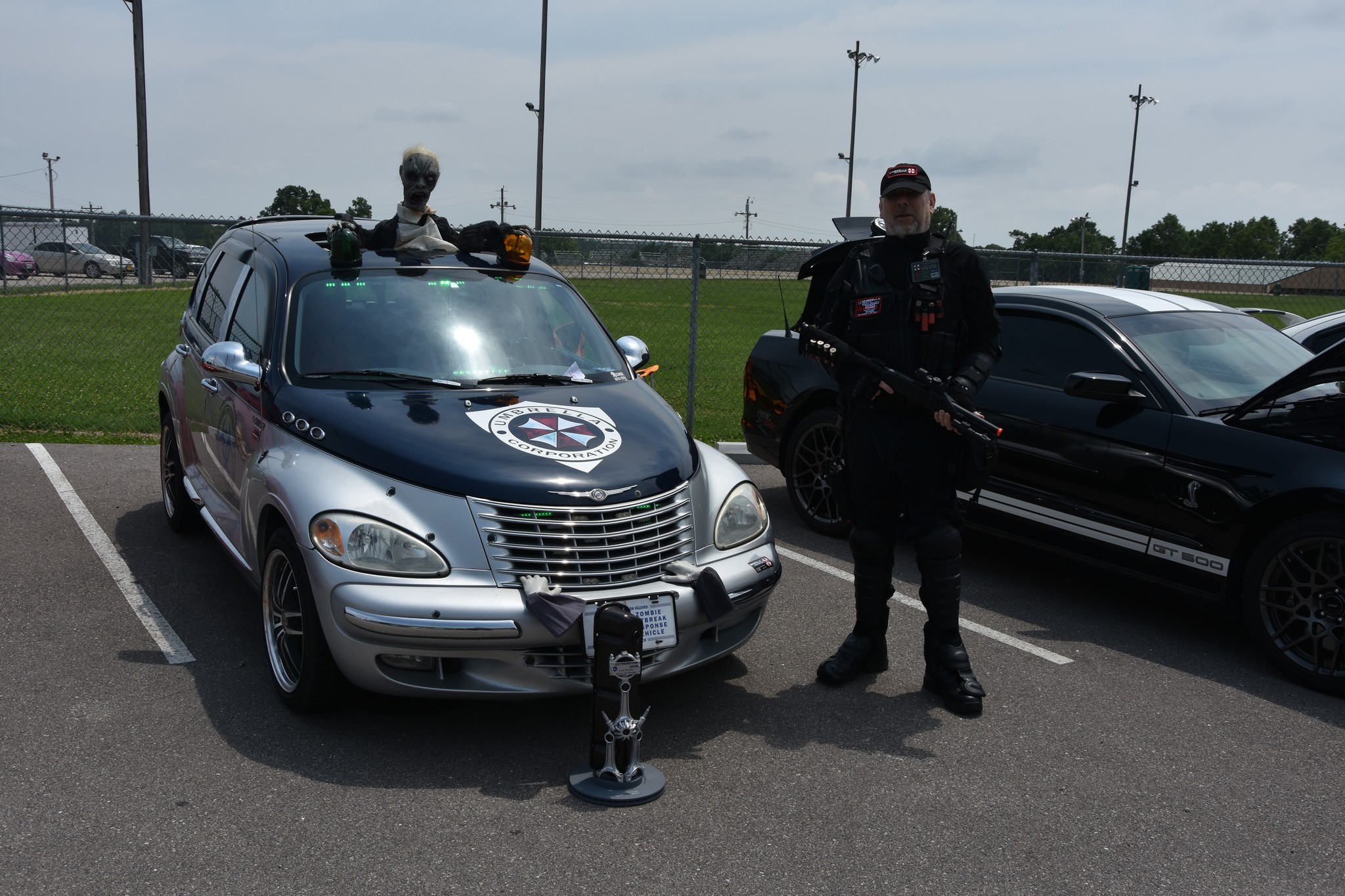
[816,634,889,681]
[923,666,983,713]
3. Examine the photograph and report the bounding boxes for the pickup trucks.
[93,235,211,279]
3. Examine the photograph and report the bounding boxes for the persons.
[812,163,996,717]
[326,148,536,255]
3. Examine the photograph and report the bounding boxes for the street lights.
[42,152,61,222]
[837,41,881,218]
[1117,83,1160,290]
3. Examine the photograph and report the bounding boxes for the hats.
[880,163,931,198]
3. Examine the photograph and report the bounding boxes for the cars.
[22,240,136,280]
[741,214,1345,704]
[0,242,38,281]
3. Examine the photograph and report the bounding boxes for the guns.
[796,321,1003,464]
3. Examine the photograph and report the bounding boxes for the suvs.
[153,214,783,716]
